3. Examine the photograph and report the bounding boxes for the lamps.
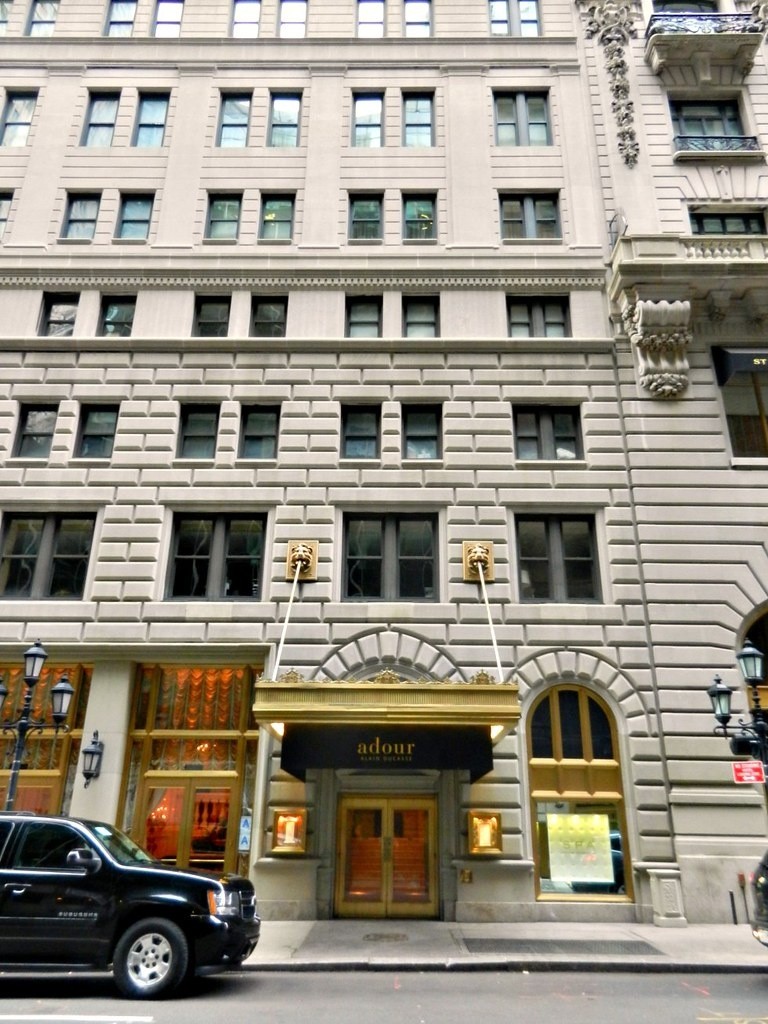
[269,809,310,856]
[79,730,105,790]
[466,811,503,856]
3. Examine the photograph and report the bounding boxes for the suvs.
[0,810,262,1002]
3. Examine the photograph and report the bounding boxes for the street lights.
[703,638,768,829]
[0,638,74,810]
